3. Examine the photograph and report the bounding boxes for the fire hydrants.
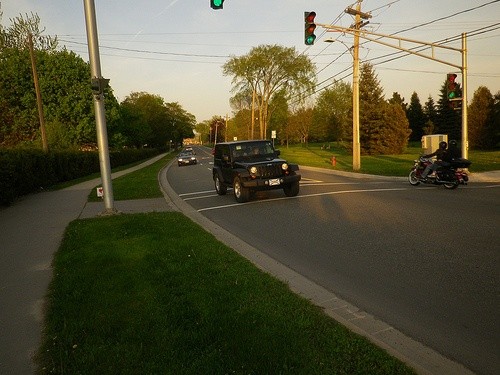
[329,154,337,168]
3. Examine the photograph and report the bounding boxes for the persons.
[420,140,447,179]
[427,139,462,178]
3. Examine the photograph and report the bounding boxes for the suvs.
[213,140,302,203]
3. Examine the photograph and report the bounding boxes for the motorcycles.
[408,155,471,189]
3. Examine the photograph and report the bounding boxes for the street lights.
[217,121,228,142]
[324,37,362,171]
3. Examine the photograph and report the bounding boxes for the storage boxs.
[453,158,471,167]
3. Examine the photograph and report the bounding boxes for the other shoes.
[428,173,435,177]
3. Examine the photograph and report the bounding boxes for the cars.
[177,151,198,167]
[186,146,193,151]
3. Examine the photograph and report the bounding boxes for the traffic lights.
[210,0,225,10]
[88,78,101,95]
[101,78,110,96]
[447,73,458,100]
[303,11,317,45]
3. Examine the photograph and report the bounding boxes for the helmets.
[439,142,447,149]
[449,140,457,147]
